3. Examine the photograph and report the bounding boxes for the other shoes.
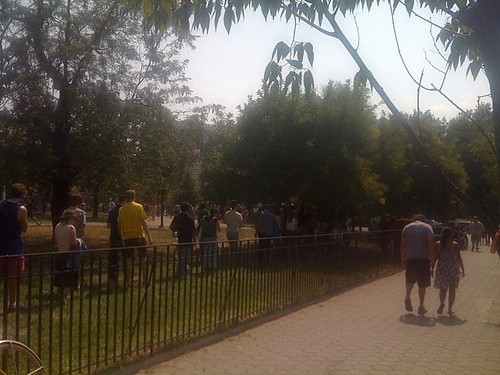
[418,307,427,313]
[476,248,478,251]
[448,310,455,314]
[436,304,445,314]
[9,304,25,311]
[142,281,150,286]
[471,248,474,251]
[127,284,132,289]
[404,299,413,311]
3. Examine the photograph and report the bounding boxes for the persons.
[24,198,41,226]
[0,182,27,314]
[52,189,367,303]
[368,213,500,319]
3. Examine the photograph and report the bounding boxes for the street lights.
[160,187,168,228]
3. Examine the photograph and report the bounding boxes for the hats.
[231,200,240,208]
[60,210,78,218]
